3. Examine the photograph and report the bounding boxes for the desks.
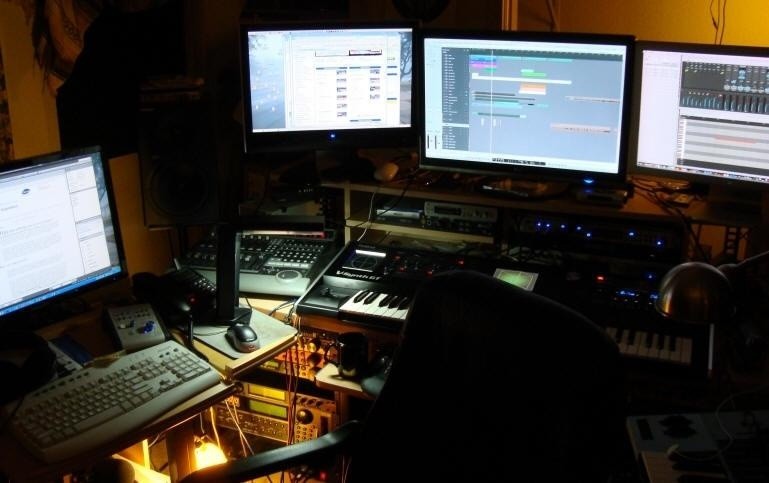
[0,339,239,483]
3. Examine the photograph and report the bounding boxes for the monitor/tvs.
[239,19,421,183]
[415,27,636,203]
[626,40,769,219]
[0,144,129,330]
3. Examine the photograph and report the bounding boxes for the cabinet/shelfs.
[191,173,752,444]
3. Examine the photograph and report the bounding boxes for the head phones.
[0,330,58,407]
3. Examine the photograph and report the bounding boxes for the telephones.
[131,266,216,330]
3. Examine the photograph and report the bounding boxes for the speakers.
[138,122,219,226]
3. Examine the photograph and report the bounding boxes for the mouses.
[373,160,400,182]
[227,323,259,353]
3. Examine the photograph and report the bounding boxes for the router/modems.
[576,186,624,207]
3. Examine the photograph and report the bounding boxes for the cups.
[323,331,369,381]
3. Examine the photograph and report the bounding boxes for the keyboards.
[5,340,220,464]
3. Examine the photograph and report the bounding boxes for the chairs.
[177,272,624,483]
